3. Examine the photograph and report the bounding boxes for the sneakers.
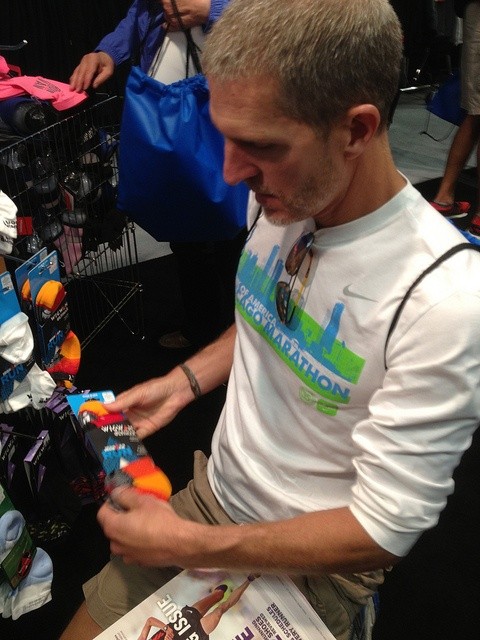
[431,199,470,220]
[467,215,480,240]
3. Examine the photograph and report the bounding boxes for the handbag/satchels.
[419,68,468,142]
[116,66,249,246]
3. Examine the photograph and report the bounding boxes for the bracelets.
[179,363,201,399]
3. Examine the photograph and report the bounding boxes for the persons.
[60,0,479,640]
[70,0,265,253]
[139,571,264,640]
[431,0,479,242]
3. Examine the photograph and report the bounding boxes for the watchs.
[163,625,168,631]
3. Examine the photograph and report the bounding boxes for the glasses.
[275,230,317,324]
